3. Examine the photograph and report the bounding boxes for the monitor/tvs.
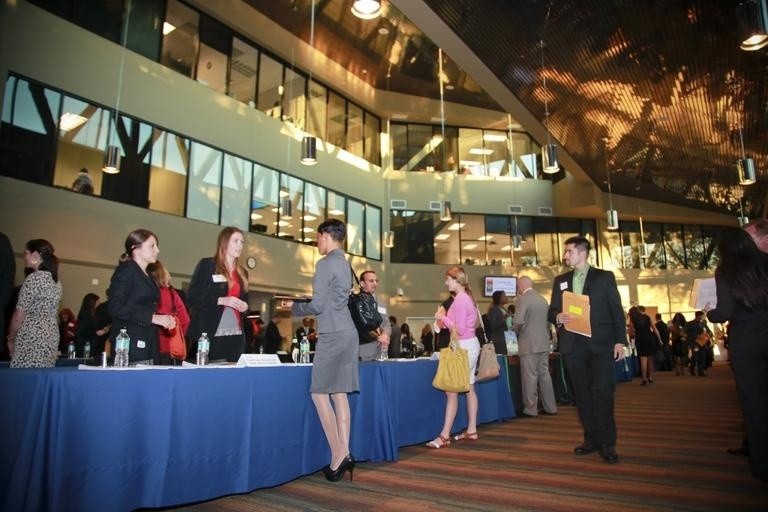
[483,276,517,297]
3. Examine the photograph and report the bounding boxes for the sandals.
[453,431,480,440]
[425,434,452,448]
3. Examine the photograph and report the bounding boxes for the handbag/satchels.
[349,263,383,333]
[475,340,500,382]
[169,286,187,360]
[432,324,470,392]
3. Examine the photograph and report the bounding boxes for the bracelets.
[7,334,18,343]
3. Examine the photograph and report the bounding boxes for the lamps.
[510,111,522,252]
[730,98,757,185]
[605,147,621,231]
[732,1,768,51]
[439,45,452,222]
[299,0,318,168]
[539,40,560,175]
[101,0,131,177]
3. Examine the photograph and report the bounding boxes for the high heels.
[323,453,355,481]
[649,378,653,384]
[641,380,647,386]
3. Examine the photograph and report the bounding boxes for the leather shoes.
[598,441,618,462]
[727,446,748,456]
[575,441,597,454]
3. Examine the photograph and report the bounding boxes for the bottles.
[67,341,76,360]
[195,332,210,367]
[83,341,90,358]
[113,329,130,368]
[290,336,310,364]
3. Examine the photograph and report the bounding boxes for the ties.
[574,272,582,293]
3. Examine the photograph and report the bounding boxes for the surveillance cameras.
[396,287,403,297]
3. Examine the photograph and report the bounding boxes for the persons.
[353,271,390,358]
[544,235,628,465]
[445,153,458,173]
[148,260,190,366]
[250,315,316,360]
[388,316,433,359]
[510,275,560,419]
[71,166,95,195]
[103,226,178,366]
[702,223,768,487]
[278,218,361,482]
[5,237,64,370]
[628,307,715,386]
[59,289,114,364]
[423,262,483,449]
[487,291,511,354]
[1,230,19,365]
[741,218,767,255]
[184,226,251,365]
